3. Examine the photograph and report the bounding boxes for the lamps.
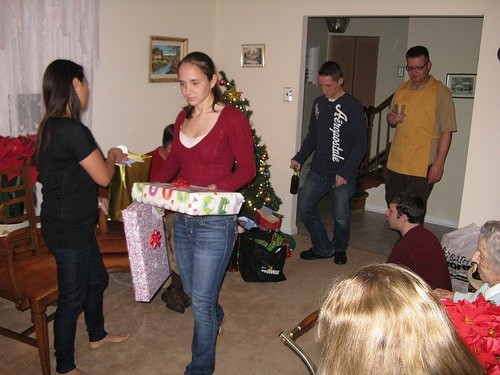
[325,17,349,33]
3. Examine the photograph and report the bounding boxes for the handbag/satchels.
[440,224,481,293]
[107,153,152,222]
[238,228,288,281]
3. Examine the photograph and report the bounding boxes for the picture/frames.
[446,73,477,99]
[241,44,265,68]
[148,35,188,82]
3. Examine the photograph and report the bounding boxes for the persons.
[315,262,487,375]
[150,124,192,313]
[154,52,256,375]
[34,58,130,375]
[386,191,500,310]
[289,60,369,265]
[384,45,457,227]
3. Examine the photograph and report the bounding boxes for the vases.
[0,172,24,224]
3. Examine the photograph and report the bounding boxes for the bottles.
[291,167,299,194]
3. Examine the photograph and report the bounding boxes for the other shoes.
[301,248,334,259]
[334,250,348,265]
[161,284,192,313]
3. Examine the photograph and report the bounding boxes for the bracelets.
[98,197,102,206]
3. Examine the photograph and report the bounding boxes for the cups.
[393,103,405,123]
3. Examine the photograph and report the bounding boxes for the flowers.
[440,294,500,375]
[0,134,40,187]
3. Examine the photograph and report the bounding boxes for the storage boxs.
[122,202,170,302]
[131,182,245,215]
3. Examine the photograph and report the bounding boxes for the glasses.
[406,61,428,71]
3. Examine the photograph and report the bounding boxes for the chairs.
[0,159,132,375]
